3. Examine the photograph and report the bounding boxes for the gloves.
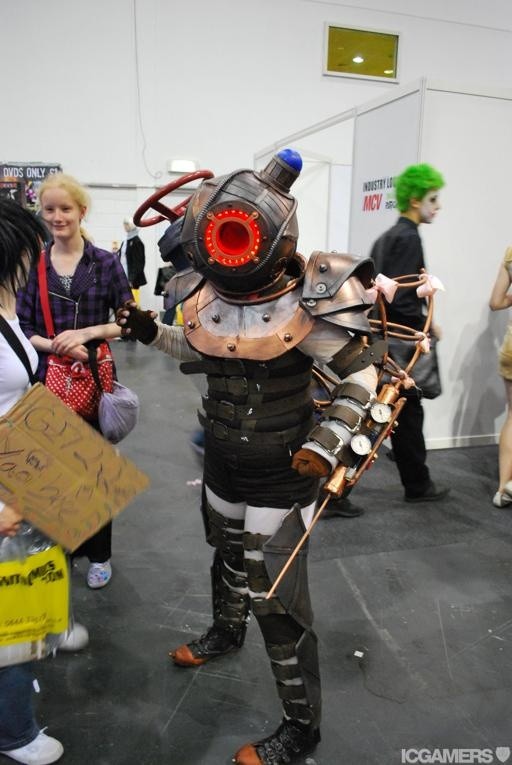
[291,448,329,477]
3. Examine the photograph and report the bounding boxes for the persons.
[16,175,135,589]
[0,197,90,765]
[116,149,378,765]
[489,247,512,508]
[113,217,147,342]
[316,163,447,518]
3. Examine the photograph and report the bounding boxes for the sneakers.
[492,492,510,508]
[58,624,91,651]
[502,478,512,502]
[86,561,113,588]
[173,620,247,665]
[234,720,321,764]
[4,726,64,764]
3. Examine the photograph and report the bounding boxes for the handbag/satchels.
[45,342,113,414]
[82,337,140,442]
[1,529,72,667]
[379,336,442,400]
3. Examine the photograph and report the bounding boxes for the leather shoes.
[316,499,364,518]
[404,484,450,502]
